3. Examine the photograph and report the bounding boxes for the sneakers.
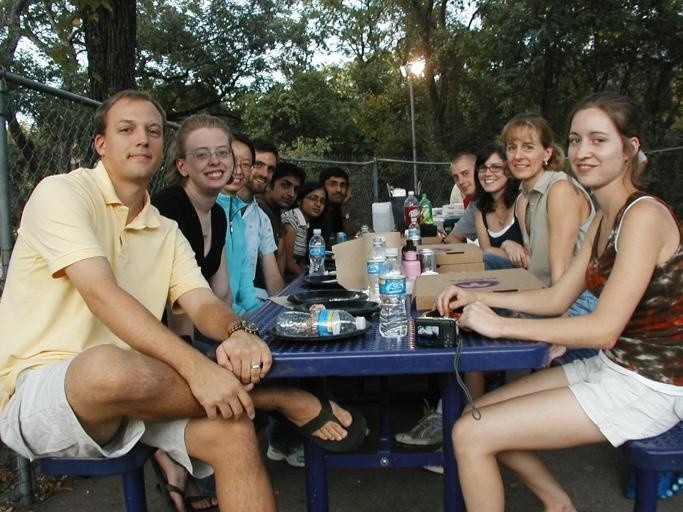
[267,400,445,473]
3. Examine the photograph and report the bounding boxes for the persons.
[146,115,236,512]
[0,90,366,512]
[451,93,683,512]
[396,114,596,475]
[445,151,479,243]
[475,143,528,268]
[217,134,353,317]
[637,150,648,177]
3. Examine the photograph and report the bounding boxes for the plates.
[293,301,380,317]
[304,273,338,286]
[420,306,520,330]
[270,318,372,341]
[286,291,368,304]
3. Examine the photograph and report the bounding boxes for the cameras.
[413,316,458,350]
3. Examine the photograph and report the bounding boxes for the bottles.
[275,310,365,337]
[377,247,407,339]
[387,184,435,295]
[360,225,370,236]
[336,232,346,244]
[366,237,389,302]
[308,228,324,277]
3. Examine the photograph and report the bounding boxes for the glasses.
[476,163,502,172]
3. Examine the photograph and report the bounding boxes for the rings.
[249,364,259,371]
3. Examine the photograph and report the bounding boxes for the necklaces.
[497,216,505,227]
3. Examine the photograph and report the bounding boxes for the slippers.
[150,458,220,512]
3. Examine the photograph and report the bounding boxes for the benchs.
[625,420,683,512]
[38,443,150,512]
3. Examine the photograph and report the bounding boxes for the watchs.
[228,319,262,338]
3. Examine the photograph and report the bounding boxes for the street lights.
[400,56,427,197]
